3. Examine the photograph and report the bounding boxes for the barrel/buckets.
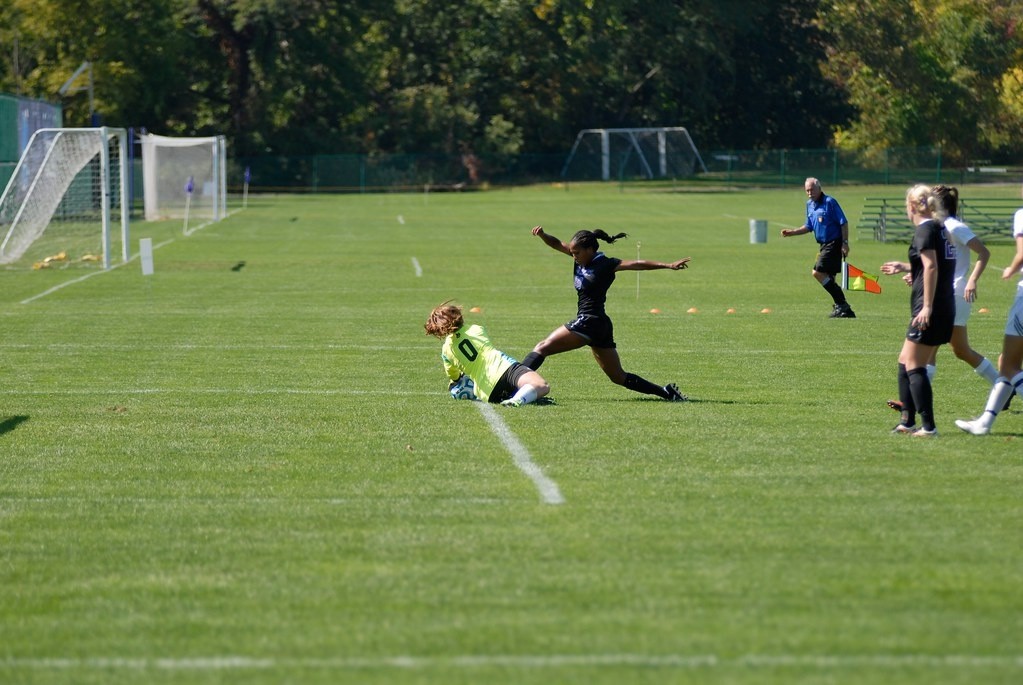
[749,220,768,243]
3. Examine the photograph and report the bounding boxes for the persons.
[523,226,691,401]
[942,205,1023,436]
[421,299,551,407]
[782,175,857,318]
[888,182,957,439]
[880,185,1014,412]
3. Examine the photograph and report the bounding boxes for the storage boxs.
[749,219,768,243]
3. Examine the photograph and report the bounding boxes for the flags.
[841,261,881,294]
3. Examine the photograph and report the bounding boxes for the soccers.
[449,376,477,401]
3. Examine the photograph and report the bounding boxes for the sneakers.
[891,424,938,436]
[886,399,903,410]
[664,383,686,401]
[1003,388,1017,410]
[829,305,855,317]
[954,420,989,436]
[501,398,520,408]
